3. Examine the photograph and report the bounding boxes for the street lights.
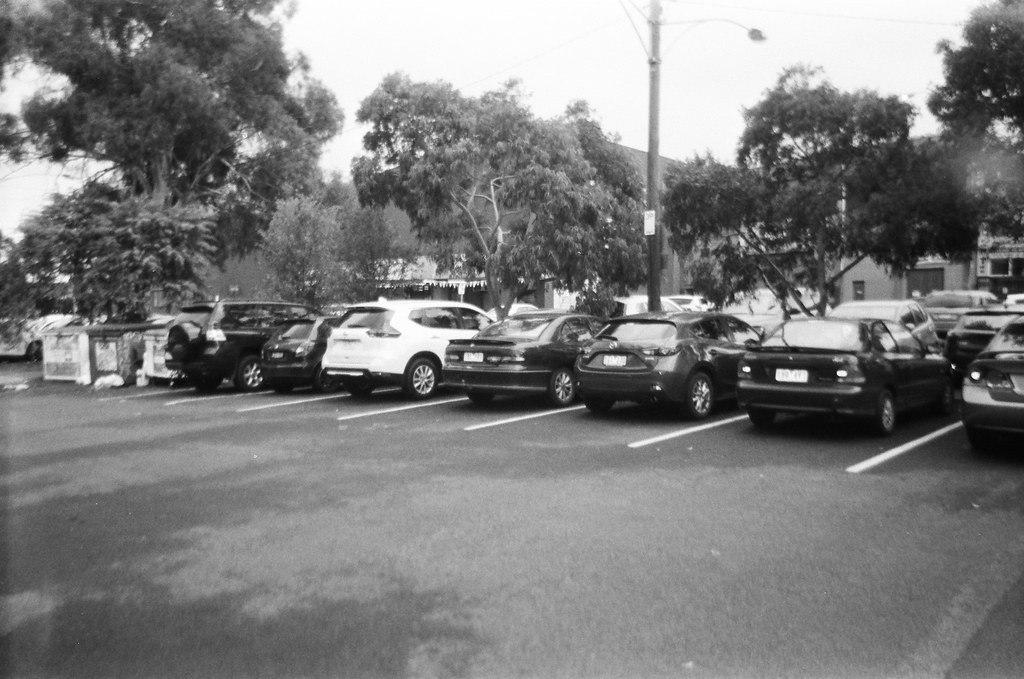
[644,0,767,314]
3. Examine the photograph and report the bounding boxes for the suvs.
[163,297,323,396]
[322,297,507,400]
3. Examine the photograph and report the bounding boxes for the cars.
[1,313,108,363]
[442,311,614,410]
[475,289,1024,367]
[258,312,341,397]
[959,312,1024,452]
[574,310,766,421]
[734,314,960,438]
